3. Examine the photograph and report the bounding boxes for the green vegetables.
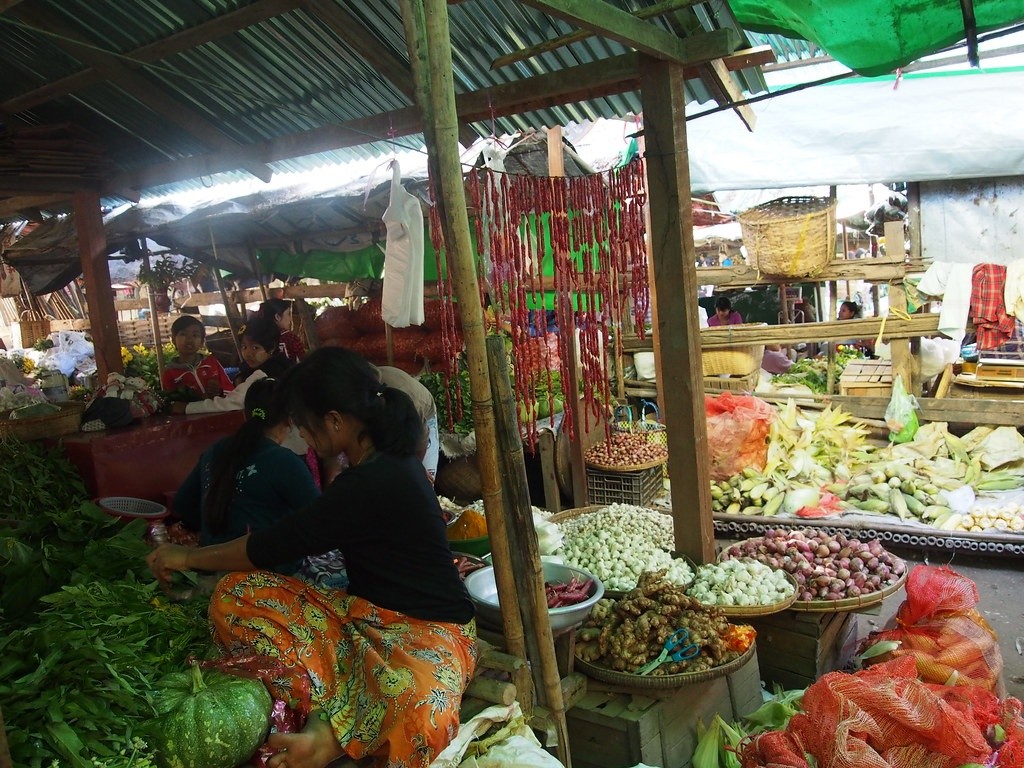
[769,345,868,399]
[418,366,587,439]
[0,426,233,768]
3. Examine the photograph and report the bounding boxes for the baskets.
[0,402,86,440]
[12,309,52,348]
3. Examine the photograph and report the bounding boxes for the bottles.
[41,368,70,401]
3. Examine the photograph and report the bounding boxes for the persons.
[171,375,321,547]
[376,366,440,483]
[262,298,306,364]
[762,342,795,374]
[162,316,234,399]
[706,296,742,326]
[832,301,861,320]
[169,318,289,414]
[235,318,294,383]
[146,347,477,765]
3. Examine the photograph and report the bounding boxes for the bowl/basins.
[464,561,604,633]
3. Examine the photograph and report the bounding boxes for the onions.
[723,528,906,601]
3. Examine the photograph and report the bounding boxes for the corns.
[710,464,1024,532]
[695,606,1024,768]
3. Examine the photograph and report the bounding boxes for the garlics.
[557,528,698,593]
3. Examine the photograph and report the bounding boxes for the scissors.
[634,628,699,676]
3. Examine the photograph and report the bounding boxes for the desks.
[36,410,249,506]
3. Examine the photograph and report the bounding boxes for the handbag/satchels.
[610,402,669,477]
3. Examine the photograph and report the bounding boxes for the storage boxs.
[586,466,667,508]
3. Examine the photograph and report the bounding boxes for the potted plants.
[140,257,199,312]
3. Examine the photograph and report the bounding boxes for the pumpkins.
[149,660,273,768]
